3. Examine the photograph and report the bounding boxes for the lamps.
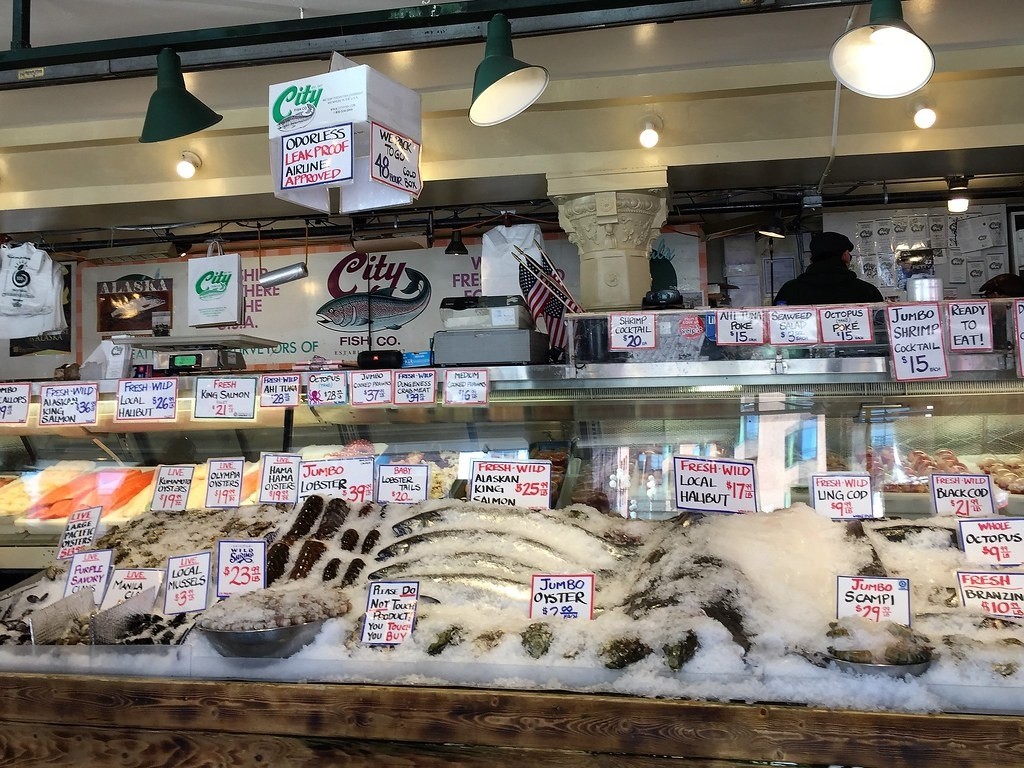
[445,230,469,255]
[137,48,223,143]
[946,176,968,212]
[828,0,937,99]
[467,13,549,128]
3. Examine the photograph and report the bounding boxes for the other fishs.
[346,504,1024,670]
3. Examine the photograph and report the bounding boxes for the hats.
[809,232,854,253]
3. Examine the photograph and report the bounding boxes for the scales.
[110,335,283,373]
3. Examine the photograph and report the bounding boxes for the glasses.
[849,253,852,261]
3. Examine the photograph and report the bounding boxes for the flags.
[518,252,585,352]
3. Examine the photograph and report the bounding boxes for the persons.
[772,232,884,306]
[978,273,1024,349]
